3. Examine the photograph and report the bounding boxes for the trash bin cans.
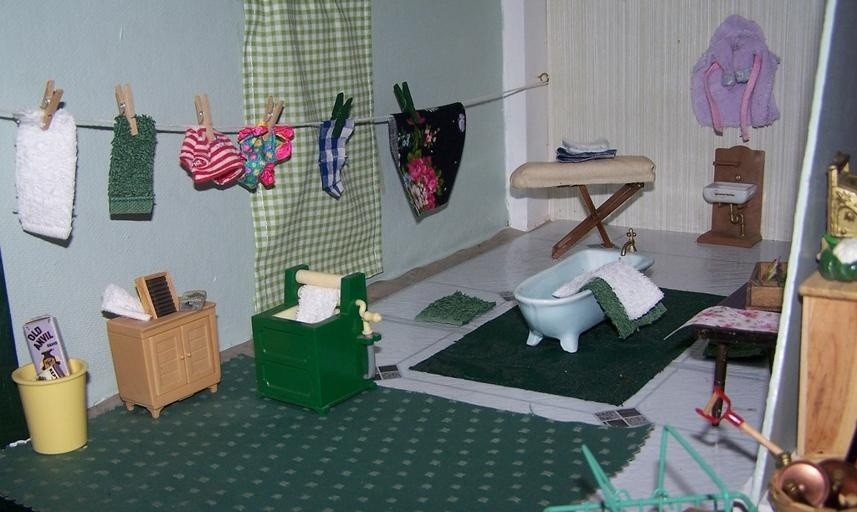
[12,358,88,455]
